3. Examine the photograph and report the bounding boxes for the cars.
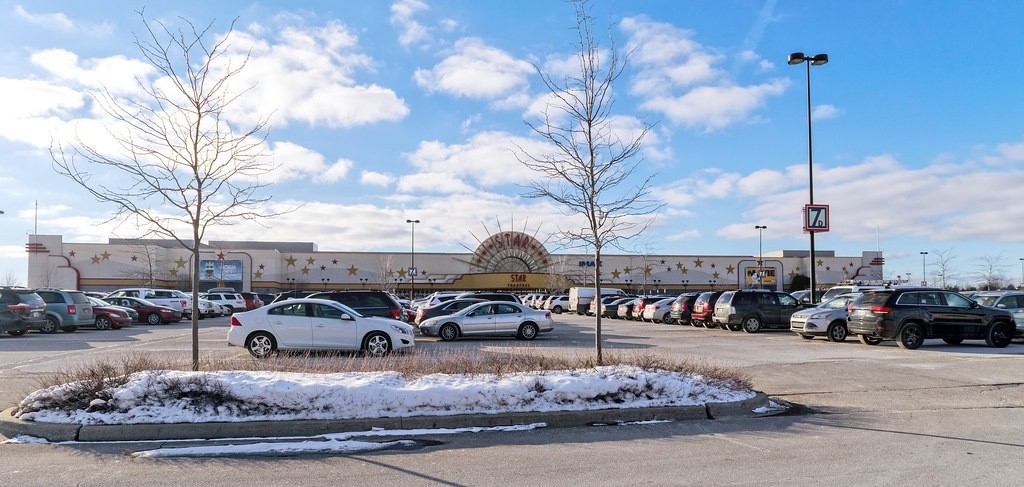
[772,282,892,307]
[948,288,1024,339]
[80,284,730,330]
[789,291,865,342]
[226,297,415,359]
[418,301,555,342]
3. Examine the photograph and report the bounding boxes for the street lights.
[1019,258,1024,288]
[625,279,633,294]
[287,278,295,291]
[394,278,403,295]
[321,278,331,290]
[407,219,421,299]
[786,52,830,305]
[754,225,767,289]
[359,278,368,289]
[920,251,929,287]
[905,272,911,285]
[428,278,436,294]
[708,279,717,290]
[653,279,661,295]
[681,279,690,290]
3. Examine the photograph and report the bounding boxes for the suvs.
[846,287,1017,350]
[0,285,49,336]
[712,289,809,334]
[28,287,96,335]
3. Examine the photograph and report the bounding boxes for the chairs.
[124,301,128,305]
[926,295,939,305]
